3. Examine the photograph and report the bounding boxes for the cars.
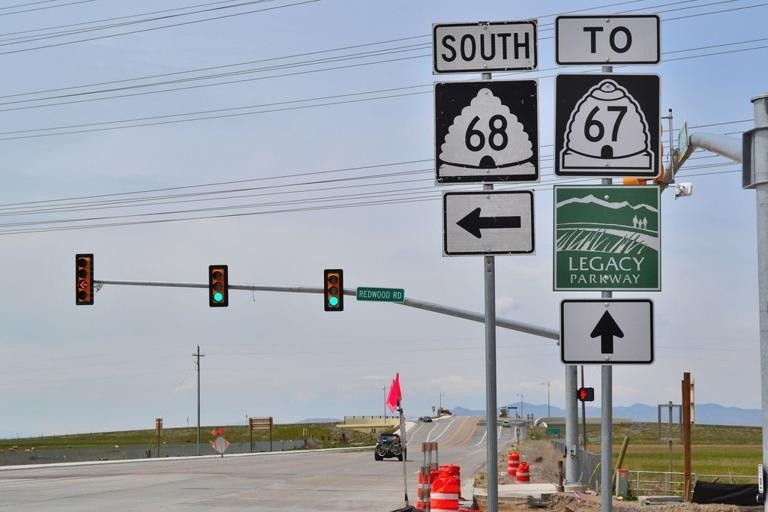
[423,416,432,422]
[503,420,510,427]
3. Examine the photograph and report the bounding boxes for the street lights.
[516,392,523,419]
[541,381,550,416]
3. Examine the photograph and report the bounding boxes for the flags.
[385,372,402,414]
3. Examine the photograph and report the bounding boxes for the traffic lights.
[75,253,94,304]
[209,265,229,307]
[621,178,647,186]
[576,387,594,401]
[325,269,344,311]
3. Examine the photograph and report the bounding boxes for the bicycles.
[378,440,401,457]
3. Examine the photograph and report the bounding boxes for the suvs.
[375,433,408,461]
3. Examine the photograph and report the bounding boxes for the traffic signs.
[357,286,404,303]
